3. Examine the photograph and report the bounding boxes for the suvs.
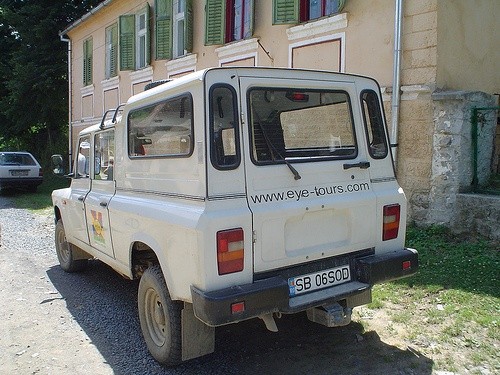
[51,65,420,367]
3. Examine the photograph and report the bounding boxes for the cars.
[0,151,44,190]
